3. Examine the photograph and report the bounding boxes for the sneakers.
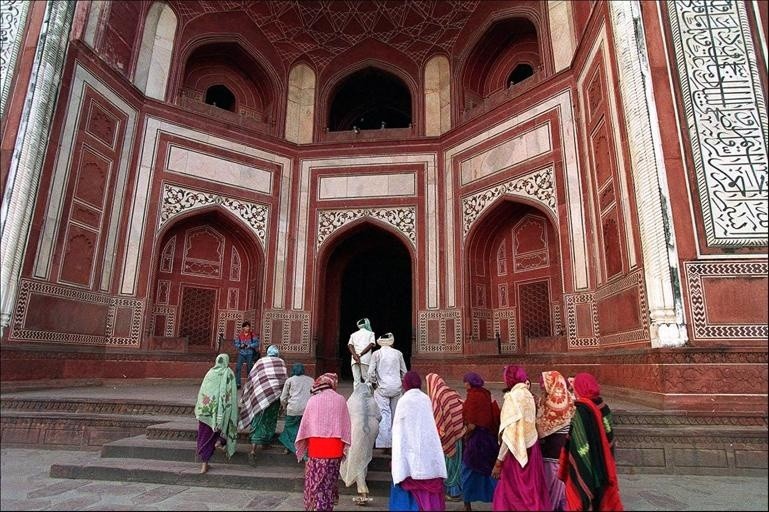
[353,496,374,505]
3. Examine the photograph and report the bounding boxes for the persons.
[349,318,408,455]
[279,362,314,461]
[194,353,238,474]
[491,364,576,512]
[559,372,624,512]
[295,373,350,512]
[425,372,463,501]
[340,384,382,507]
[232,323,259,390]
[391,372,449,512]
[238,345,289,468]
[459,372,501,512]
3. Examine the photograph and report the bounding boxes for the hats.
[464,372,485,387]
[402,371,422,390]
[573,372,601,398]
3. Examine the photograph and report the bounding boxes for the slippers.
[199,443,257,474]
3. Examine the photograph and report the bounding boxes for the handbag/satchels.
[251,350,261,363]
